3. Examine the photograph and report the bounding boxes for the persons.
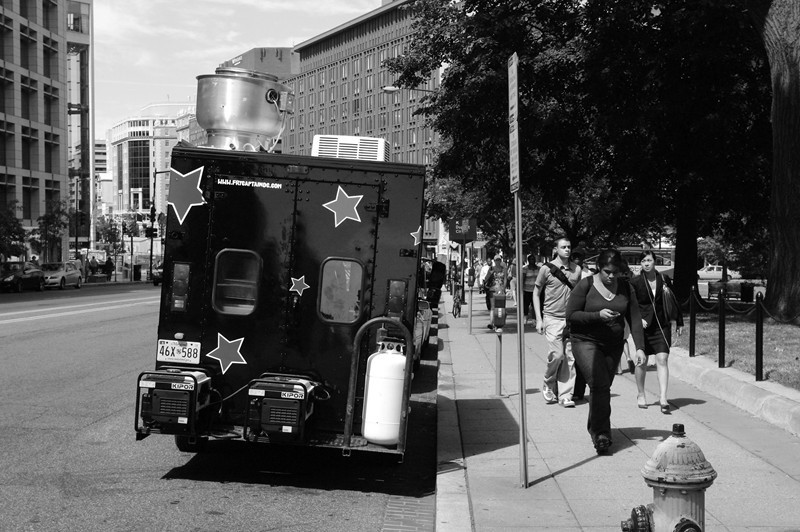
[464,248,543,331]
[566,250,647,457]
[629,249,684,414]
[75,255,97,279]
[552,247,635,399]
[29,256,39,267]
[105,257,113,281]
[533,238,582,407]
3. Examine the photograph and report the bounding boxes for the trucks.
[134,66,448,468]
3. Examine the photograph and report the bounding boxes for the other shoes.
[524,315,529,325]
[542,383,554,400]
[559,398,575,407]
[615,371,622,375]
[487,324,493,329]
[531,319,536,328]
[627,358,635,374]
[593,433,610,450]
[571,395,580,401]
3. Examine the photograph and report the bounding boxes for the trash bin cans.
[134,265,142,281]
[740,283,754,302]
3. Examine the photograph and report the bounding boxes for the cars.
[577,249,674,290]
[0,261,46,293]
[697,265,742,280]
[39,261,82,290]
[152,268,163,286]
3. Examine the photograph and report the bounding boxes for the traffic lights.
[153,228,158,238]
[144,228,151,238]
[150,207,157,222]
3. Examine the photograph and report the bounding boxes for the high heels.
[659,400,669,412]
[637,396,647,408]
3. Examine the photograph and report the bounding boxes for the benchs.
[708,281,741,300]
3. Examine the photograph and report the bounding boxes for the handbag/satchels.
[661,274,678,320]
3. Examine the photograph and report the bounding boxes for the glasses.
[529,258,535,260]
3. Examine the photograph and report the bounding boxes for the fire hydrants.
[617,421,719,531]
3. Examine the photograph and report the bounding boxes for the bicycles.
[452,282,463,318]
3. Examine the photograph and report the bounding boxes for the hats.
[32,256,36,259]
[494,255,501,260]
